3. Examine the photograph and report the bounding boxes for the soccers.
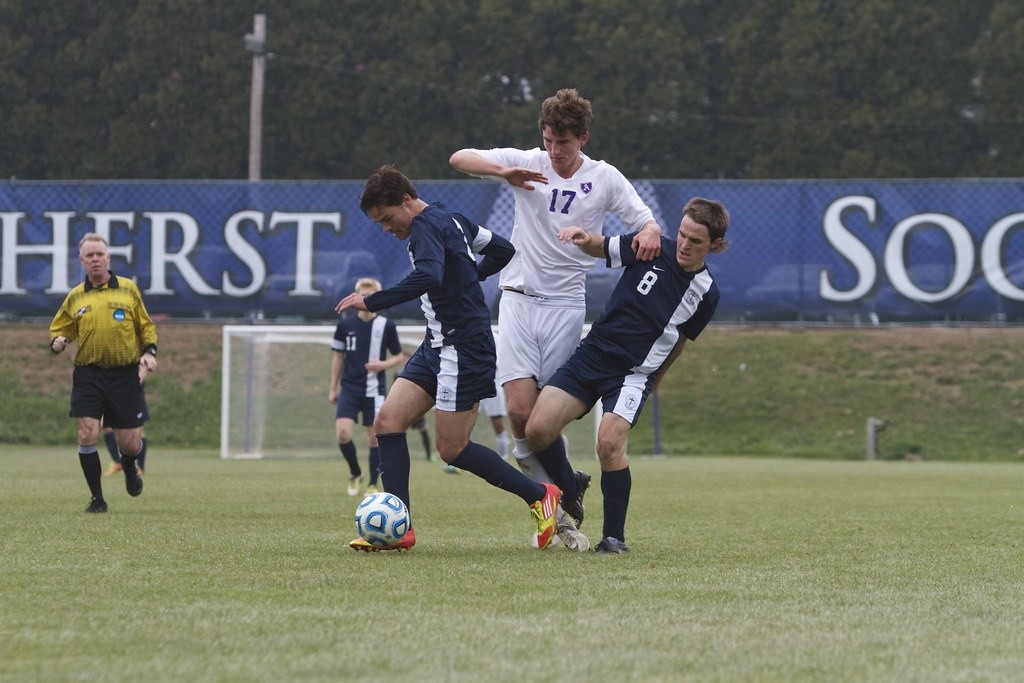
[354,491,412,550]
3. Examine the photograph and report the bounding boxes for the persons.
[49,233,158,513]
[329,90,731,554]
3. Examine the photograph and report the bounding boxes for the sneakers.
[120,455,143,496]
[595,536,630,553]
[529,481,563,550]
[104,459,121,476]
[554,512,590,552]
[560,469,592,530]
[85,495,107,512]
[441,462,464,474]
[364,484,378,497]
[349,525,416,552]
[347,471,365,496]
